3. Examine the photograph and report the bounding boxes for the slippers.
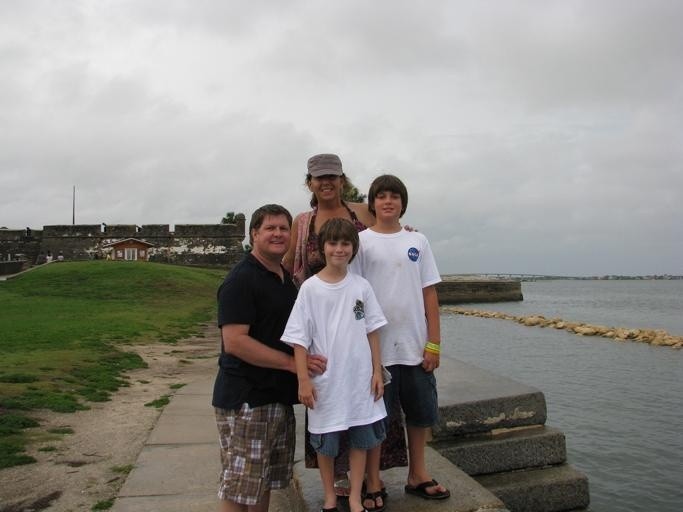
[361,488,387,511]
[404,478,450,500]
[334,476,350,497]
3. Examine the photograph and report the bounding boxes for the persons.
[345,175,449,511]
[210,204,327,510]
[280,219,389,511]
[280,154,420,496]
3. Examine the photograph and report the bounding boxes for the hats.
[307,154,343,177]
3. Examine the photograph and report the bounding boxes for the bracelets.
[424,341,440,355]
